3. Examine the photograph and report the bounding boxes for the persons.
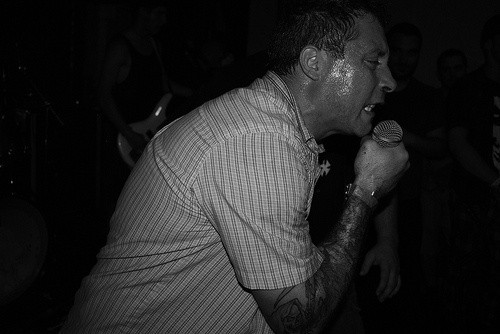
[59,1,411,334]
[1,0,191,334]
[192,0,500,334]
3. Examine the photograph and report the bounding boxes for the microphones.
[371,120,404,148]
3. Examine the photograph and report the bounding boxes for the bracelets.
[344,181,379,210]
